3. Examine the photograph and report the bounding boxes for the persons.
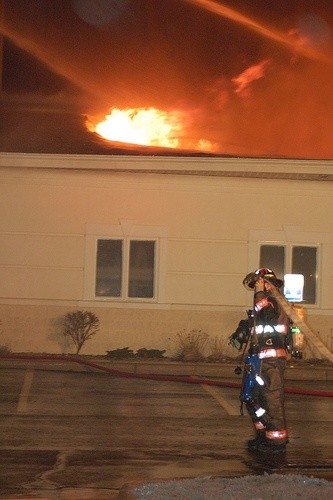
[231,267,301,448]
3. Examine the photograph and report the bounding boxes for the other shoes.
[247,433,266,446]
[254,440,286,453]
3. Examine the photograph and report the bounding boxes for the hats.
[248,268,284,288]
